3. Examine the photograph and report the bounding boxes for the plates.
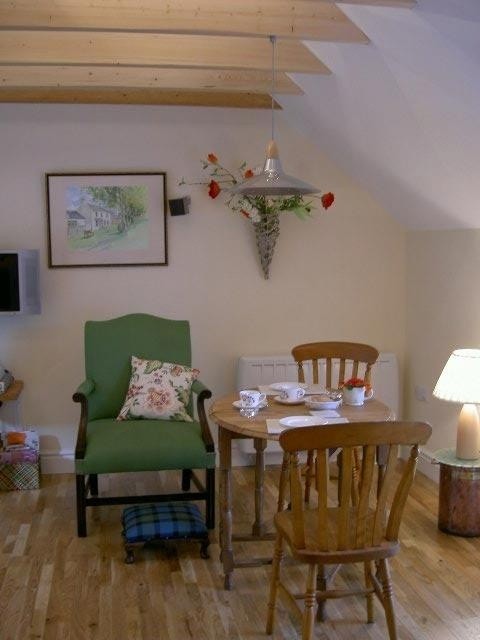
[274,396,303,405]
[233,400,269,409]
[278,415,328,426]
[270,382,310,391]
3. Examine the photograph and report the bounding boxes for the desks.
[209,387,395,592]
[432,447,480,538]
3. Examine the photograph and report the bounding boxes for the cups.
[279,386,304,400]
[240,390,266,405]
[342,385,373,406]
[240,407,259,423]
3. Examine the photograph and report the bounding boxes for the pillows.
[116,355,200,423]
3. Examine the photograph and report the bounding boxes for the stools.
[120,502,210,564]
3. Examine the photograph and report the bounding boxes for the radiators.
[236,353,399,456]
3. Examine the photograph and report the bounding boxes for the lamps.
[432,349,480,460]
[231,37,322,196]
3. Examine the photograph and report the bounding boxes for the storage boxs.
[0,429,41,491]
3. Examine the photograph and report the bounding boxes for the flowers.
[179,153,335,234]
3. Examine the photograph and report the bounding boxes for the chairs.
[278,342,380,512]
[265,421,433,640]
[72,312,215,537]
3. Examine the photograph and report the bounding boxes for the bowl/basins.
[304,396,342,408]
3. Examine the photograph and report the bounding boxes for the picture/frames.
[45,171,169,268]
